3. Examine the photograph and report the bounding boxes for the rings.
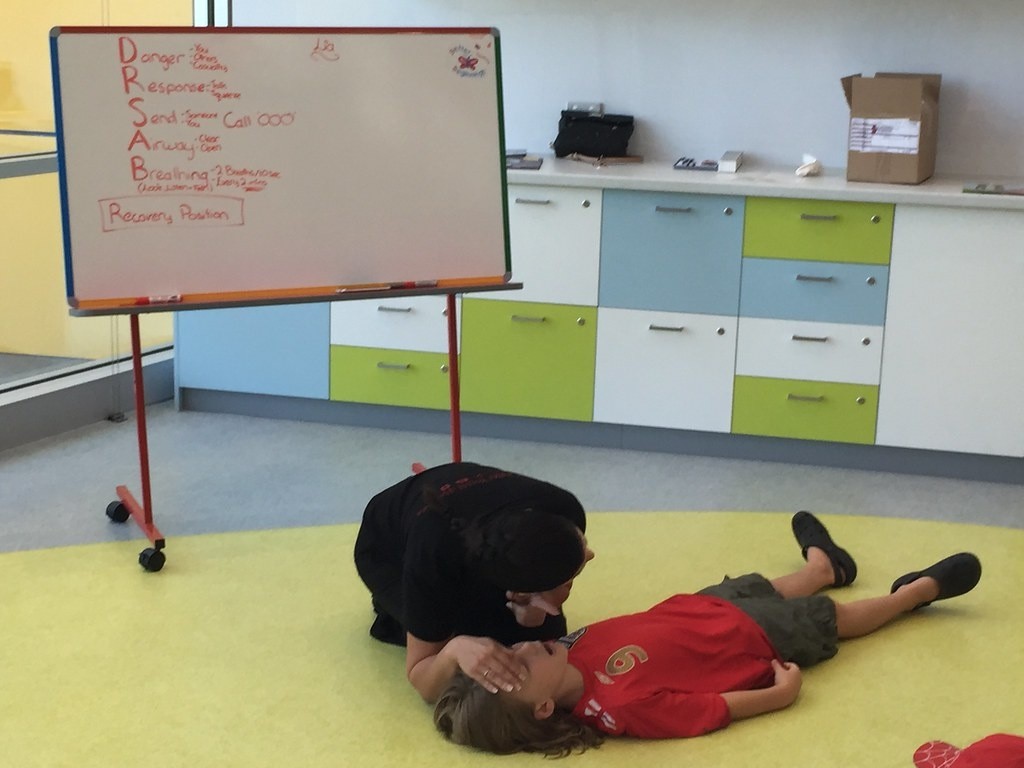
[483,668,490,677]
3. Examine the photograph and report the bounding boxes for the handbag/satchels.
[554,111,634,157]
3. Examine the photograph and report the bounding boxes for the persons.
[355,461,594,646]
[435,511,982,761]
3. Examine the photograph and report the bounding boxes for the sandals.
[891,553,981,611]
[791,510,857,586]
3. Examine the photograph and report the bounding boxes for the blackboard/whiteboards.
[48,24,514,308]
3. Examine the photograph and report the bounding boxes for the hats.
[913,733,1024,768]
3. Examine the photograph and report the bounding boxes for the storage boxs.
[840,72,942,184]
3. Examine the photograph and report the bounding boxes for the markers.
[390,279,439,289]
[134,294,184,305]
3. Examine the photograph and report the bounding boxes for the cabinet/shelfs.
[173,154,1024,485]
